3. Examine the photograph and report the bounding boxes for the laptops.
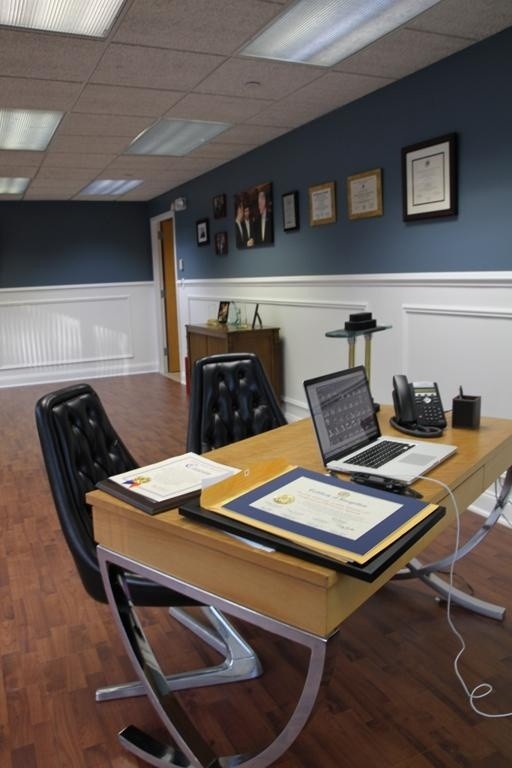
[304,365,458,485]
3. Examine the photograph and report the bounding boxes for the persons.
[243,205,258,245]
[253,191,273,244]
[234,203,254,246]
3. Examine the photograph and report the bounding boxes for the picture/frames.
[400,134,458,228]
[281,189,300,234]
[345,165,384,221]
[251,302,263,327]
[217,300,230,323]
[194,217,210,247]
[305,178,338,227]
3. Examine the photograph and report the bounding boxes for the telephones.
[392,375,447,430]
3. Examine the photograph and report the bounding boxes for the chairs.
[28,382,268,704]
[184,352,287,460]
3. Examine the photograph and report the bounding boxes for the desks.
[326,312,390,381]
[84,371,512,767]
[177,323,282,406]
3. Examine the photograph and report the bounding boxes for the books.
[95,450,245,516]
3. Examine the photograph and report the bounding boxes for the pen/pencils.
[459,385,464,400]
